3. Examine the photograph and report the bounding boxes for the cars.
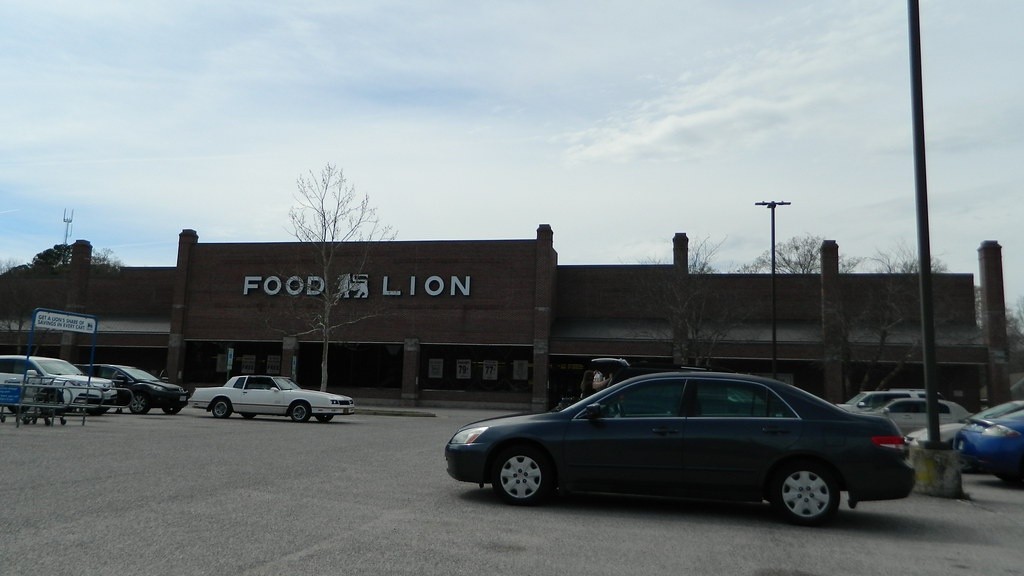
[833,385,1023,490]
[187,374,355,422]
[443,369,920,523]
[73,360,188,417]
[0,351,117,415]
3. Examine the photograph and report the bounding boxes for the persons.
[580,370,595,402]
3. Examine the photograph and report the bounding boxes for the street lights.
[754,199,793,377]
